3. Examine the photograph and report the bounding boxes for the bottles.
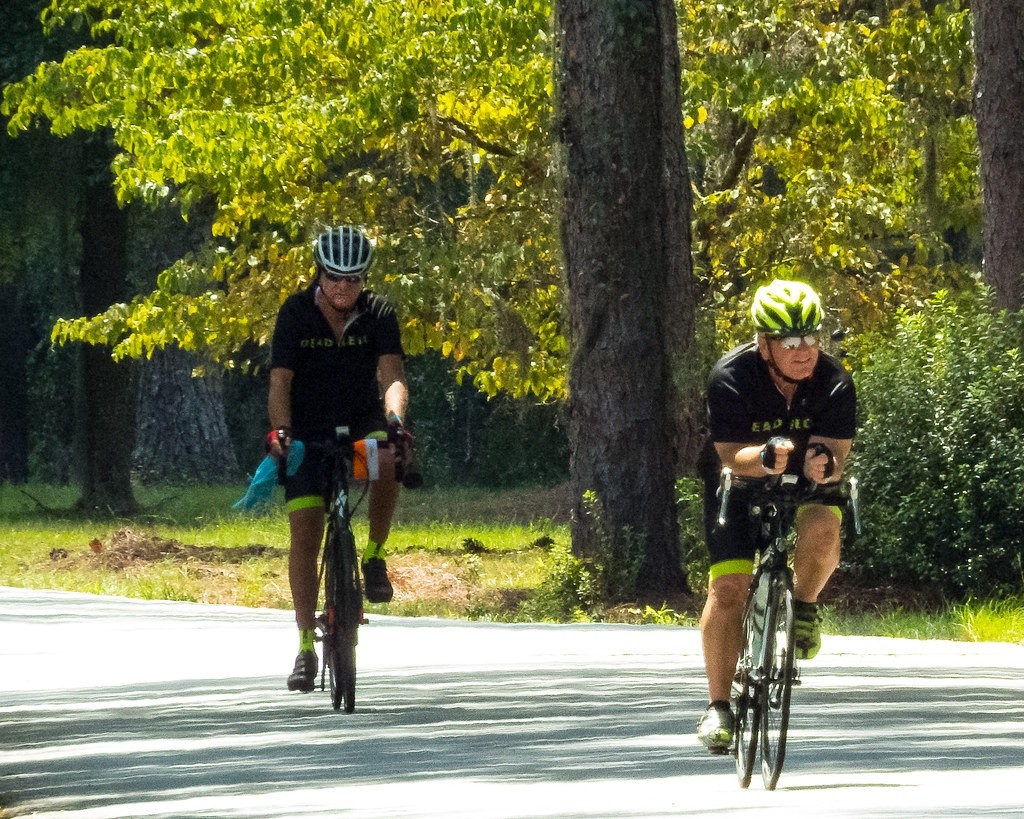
[752,600,767,666]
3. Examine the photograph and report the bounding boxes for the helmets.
[314,226,372,275]
[751,279,821,338]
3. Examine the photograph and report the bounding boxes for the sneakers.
[697,709,734,746]
[794,609,822,659]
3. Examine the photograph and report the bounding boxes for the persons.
[695,281,856,747]
[266,226,409,693]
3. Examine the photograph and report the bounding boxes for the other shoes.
[361,557,393,603]
[287,651,318,691]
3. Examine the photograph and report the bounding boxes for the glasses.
[322,268,361,283]
[780,337,816,349]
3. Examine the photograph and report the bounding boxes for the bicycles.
[717,442,864,790]
[277,415,408,713]
